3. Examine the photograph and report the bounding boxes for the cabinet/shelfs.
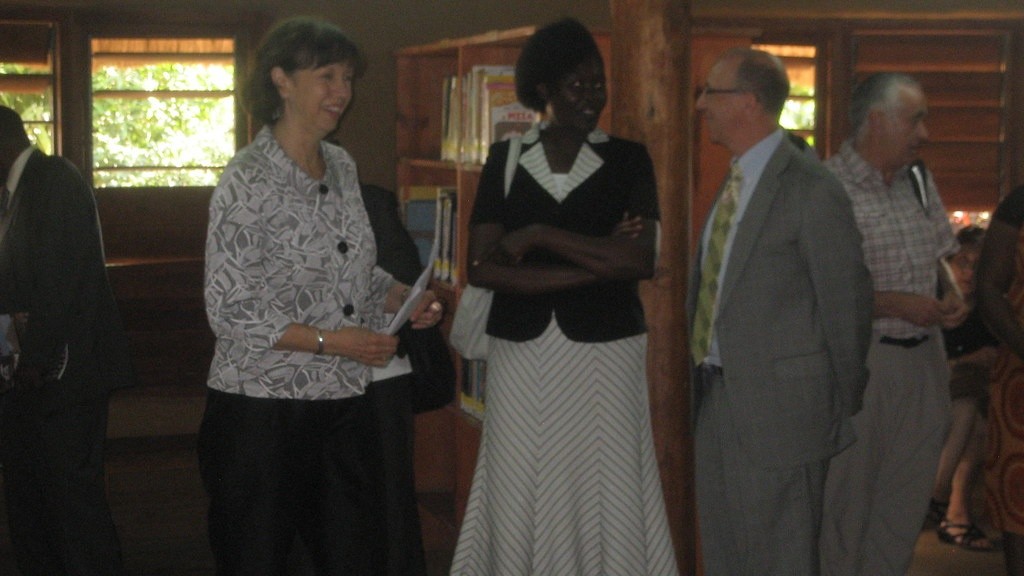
[394,25,615,576]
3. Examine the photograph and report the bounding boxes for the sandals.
[937,516,993,550]
[920,499,947,527]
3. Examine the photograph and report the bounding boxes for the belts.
[701,364,722,376]
[882,334,929,349]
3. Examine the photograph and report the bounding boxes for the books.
[439,65,538,165]
[460,355,487,421]
[400,186,459,284]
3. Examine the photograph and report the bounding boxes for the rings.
[431,302,441,310]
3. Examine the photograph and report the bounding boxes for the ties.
[689,164,737,366]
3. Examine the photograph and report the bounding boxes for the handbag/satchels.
[450,282,494,360]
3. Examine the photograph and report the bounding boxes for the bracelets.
[316,329,324,354]
[401,289,408,305]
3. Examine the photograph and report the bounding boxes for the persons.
[194,16,455,576]
[822,70,1024,576]
[686,50,872,576]
[0,107,132,576]
[447,18,682,576]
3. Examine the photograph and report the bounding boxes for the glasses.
[704,87,741,97]
